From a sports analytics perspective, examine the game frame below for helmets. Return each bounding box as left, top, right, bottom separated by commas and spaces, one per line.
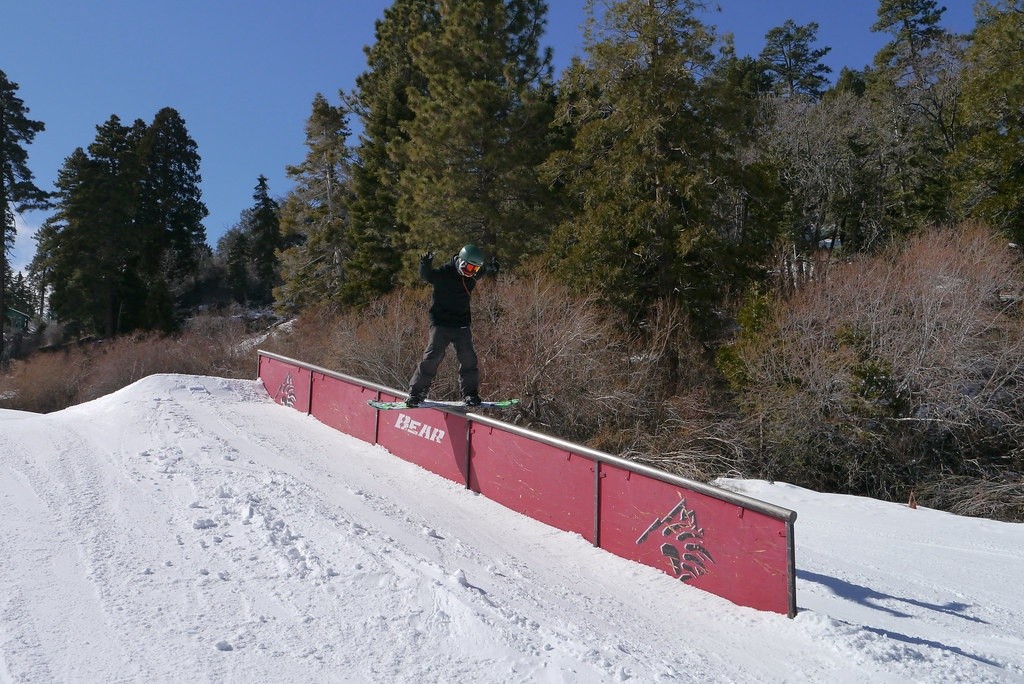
459, 244, 484, 267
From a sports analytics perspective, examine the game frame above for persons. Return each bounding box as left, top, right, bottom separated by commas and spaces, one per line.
405, 244, 500, 407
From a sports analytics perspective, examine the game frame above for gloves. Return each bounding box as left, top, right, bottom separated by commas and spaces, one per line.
420, 252, 437, 264
486, 257, 498, 277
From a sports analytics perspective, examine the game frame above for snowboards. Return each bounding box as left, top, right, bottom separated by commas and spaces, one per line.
367, 399, 520, 412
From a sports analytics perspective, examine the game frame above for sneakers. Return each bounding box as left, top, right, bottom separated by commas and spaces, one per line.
463, 391, 480, 407
407, 395, 423, 407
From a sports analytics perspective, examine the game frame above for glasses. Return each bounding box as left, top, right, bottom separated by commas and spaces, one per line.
465, 263, 480, 273
